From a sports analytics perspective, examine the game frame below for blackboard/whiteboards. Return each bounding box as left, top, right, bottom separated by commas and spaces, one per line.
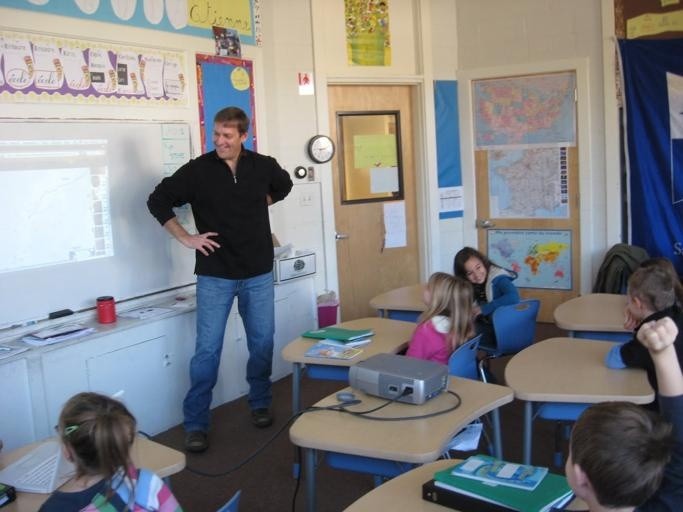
2, 118, 203, 333
268, 184, 328, 299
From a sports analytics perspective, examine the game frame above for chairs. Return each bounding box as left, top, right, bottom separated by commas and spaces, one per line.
216, 489, 246, 512
471, 299, 541, 383
448, 332, 485, 381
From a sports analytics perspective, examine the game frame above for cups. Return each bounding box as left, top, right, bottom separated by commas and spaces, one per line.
97, 296, 115, 323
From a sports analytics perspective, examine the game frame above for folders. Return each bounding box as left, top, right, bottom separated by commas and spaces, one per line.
422, 478, 577, 512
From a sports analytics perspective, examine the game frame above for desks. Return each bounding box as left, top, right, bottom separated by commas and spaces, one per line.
555, 293, 643, 342
280, 317, 421, 483
341, 460, 593, 511
2, 434, 187, 511
506, 336, 654, 468
290, 374, 516, 512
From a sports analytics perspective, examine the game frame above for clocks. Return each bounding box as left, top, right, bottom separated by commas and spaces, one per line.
308, 137, 337, 165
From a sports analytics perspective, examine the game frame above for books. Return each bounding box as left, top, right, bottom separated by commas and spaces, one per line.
297, 322, 379, 360
0, 482, 15, 509
420, 450, 576, 511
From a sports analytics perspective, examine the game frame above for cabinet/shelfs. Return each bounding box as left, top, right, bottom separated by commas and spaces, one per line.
0, 279, 321, 459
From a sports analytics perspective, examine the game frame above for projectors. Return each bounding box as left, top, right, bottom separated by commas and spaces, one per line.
348, 352, 449, 405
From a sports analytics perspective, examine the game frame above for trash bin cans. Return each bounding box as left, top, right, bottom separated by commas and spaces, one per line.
315, 289, 339, 328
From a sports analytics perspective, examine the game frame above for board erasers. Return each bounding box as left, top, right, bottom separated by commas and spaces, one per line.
49, 310, 75, 320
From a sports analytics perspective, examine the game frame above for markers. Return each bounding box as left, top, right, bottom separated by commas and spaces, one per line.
1, 348, 14, 352
12, 320, 41, 329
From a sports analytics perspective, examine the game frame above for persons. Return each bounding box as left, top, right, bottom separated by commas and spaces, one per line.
603, 258, 680, 389
547, 314, 682, 512
36, 389, 183, 512
146, 104, 294, 451
453, 244, 522, 320
406, 271, 476, 366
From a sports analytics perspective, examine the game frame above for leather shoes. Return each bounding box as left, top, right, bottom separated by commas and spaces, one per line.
252, 408, 272, 427
185, 429, 208, 451
